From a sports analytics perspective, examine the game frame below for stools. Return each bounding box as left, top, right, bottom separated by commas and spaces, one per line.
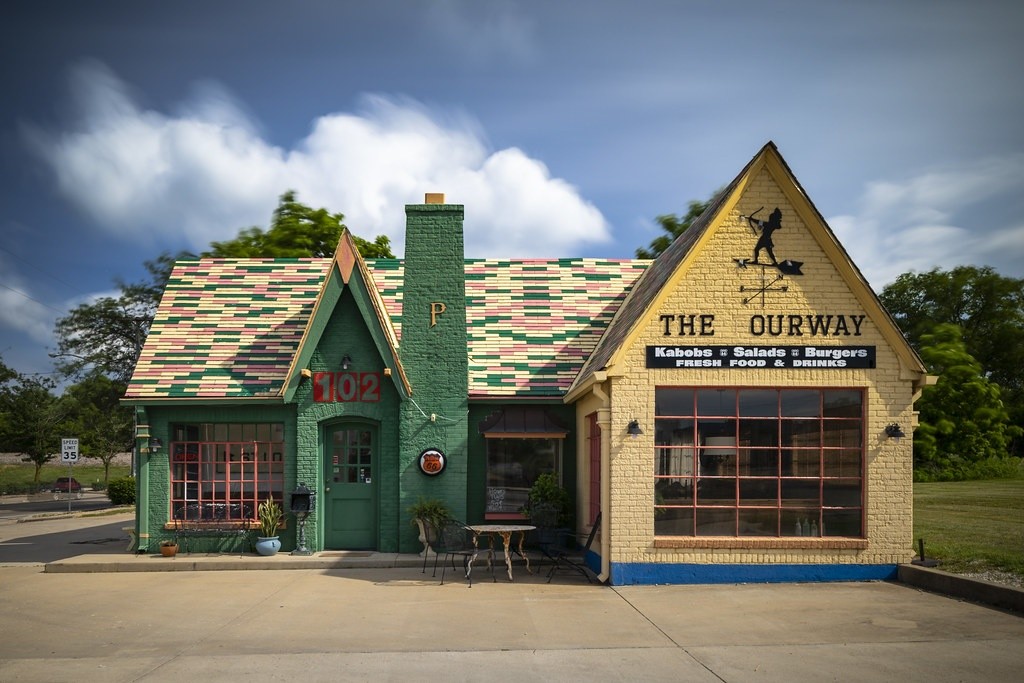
122, 526, 136, 552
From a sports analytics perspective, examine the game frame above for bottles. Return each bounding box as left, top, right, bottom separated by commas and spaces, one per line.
811, 520, 818, 536
795, 517, 801, 536
802, 517, 810, 535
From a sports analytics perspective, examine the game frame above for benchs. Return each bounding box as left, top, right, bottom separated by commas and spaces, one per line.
173, 504, 254, 559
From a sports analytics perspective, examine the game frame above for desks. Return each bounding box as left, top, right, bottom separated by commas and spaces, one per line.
461, 525, 536, 581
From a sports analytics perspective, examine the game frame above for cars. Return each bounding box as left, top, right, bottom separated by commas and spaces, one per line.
55, 478, 81, 492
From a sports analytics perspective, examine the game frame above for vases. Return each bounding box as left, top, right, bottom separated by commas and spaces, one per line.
159, 540, 177, 556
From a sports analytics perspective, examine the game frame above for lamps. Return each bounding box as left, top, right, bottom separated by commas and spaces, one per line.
626, 420, 644, 438
149, 437, 162, 452
340, 354, 353, 369
886, 423, 905, 443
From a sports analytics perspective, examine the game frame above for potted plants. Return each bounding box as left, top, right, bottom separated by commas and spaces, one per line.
255, 496, 290, 556
525, 469, 572, 554
404, 492, 455, 557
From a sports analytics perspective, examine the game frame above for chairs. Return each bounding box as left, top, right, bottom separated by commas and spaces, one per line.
417, 511, 602, 588
